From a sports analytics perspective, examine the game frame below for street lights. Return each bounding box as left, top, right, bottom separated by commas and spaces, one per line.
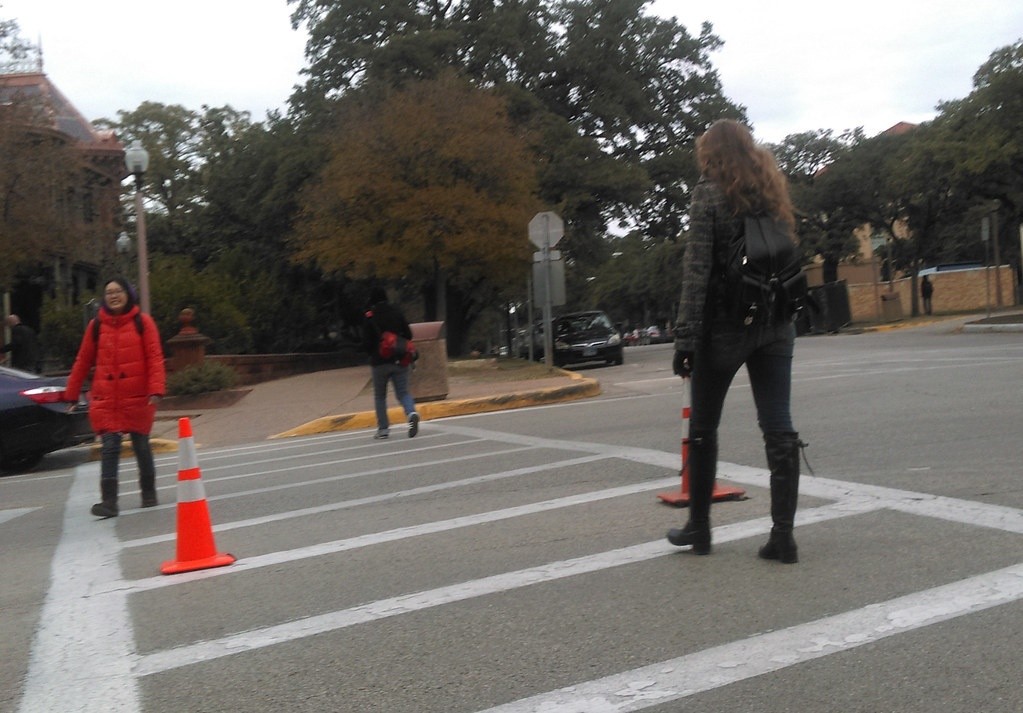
124, 147, 150, 315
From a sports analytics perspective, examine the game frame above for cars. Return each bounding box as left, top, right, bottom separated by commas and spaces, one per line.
514, 310, 674, 368
0, 365, 95, 476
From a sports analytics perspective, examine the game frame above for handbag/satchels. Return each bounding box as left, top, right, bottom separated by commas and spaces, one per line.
379, 332, 419, 368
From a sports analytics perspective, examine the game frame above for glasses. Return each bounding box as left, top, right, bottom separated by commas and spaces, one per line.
106, 289, 125, 296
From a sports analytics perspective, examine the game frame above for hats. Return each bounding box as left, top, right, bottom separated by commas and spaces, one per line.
369, 288, 387, 304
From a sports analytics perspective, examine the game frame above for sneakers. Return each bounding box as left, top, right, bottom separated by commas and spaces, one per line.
408, 412, 419, 439
374, 428, 390, 439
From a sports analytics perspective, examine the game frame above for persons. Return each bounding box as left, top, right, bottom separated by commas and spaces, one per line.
921, 275, 933, 314
359, 287, 419, 439
663, 118, 818, 563
0, 314, 45, 374
62, 278, 171, 514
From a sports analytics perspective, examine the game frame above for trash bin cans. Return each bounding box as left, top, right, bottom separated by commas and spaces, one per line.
881, 291, 903, 323
406, 321, 449, 402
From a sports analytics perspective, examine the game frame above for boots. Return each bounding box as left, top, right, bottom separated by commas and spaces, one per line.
667, 432, 717, 554
758, 432, 814, 564
91, 478, 119, 516
140, 480, 158, 508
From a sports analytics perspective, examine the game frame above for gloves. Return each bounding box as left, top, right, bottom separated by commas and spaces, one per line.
672, 349, 694, 380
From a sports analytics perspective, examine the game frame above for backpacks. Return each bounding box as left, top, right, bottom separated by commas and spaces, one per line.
724, 210, 810, 331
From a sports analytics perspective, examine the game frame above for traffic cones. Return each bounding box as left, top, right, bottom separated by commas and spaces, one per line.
160, 417, 236, 575
657, 376, 746, 506
